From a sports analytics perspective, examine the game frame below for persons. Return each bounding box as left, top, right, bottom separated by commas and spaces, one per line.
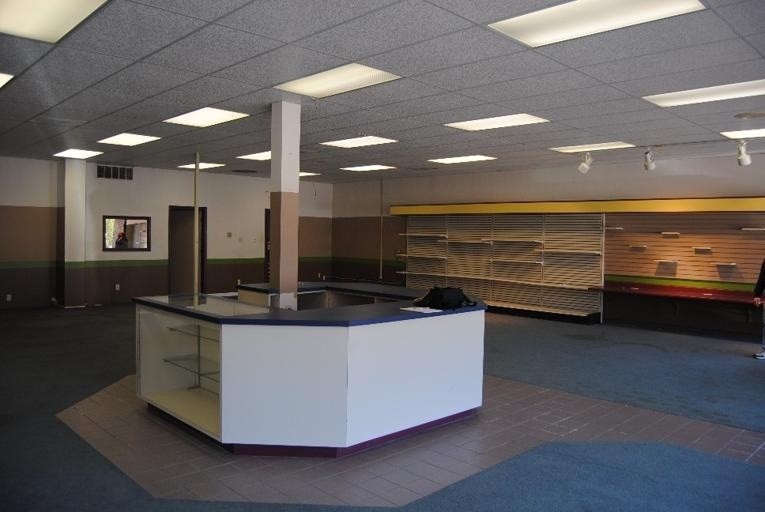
114, 232, 128, 248
749, 257, 765, 360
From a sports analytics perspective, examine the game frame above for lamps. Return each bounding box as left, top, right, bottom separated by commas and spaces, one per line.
577, 139, 764, 175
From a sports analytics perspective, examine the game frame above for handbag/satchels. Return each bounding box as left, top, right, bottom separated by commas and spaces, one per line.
413, 288, 464, 310
